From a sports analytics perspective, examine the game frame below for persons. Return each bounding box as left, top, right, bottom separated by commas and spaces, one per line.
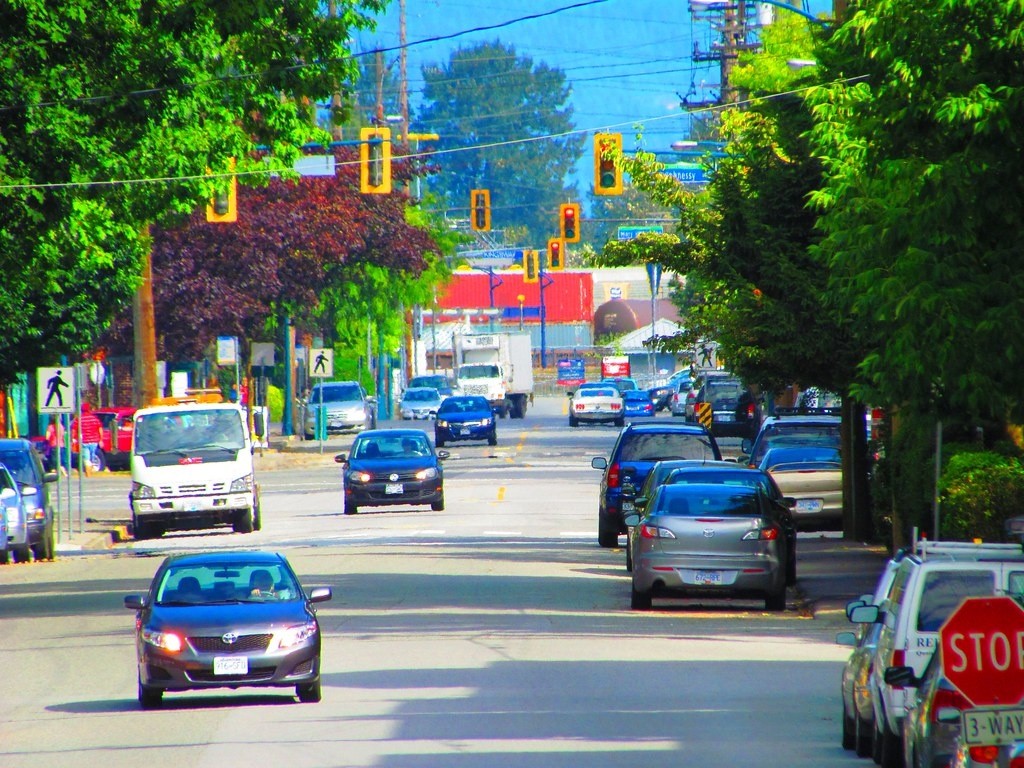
227, 376, 249, 410
396, 439, 423, 457
46, 413, 69, 475
71, 402, 104, 478
250, 572, 280, 601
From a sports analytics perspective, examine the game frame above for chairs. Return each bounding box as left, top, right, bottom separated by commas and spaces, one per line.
250, 570, 276, 594
4, 455, 27, 483
668, 496, 689, 516
178, 576, 204, 599
366, 444, 382, 458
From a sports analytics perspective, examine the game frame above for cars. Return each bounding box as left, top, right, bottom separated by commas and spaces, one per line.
432, 398, 498, 447
399, 373, 456, 420
621, 457, 798, 611
1, 440, 60, 564
649, 368, 847, 530
885, 643, 1024, 768
567, 378, 673, 427
303, 381, 378, 439
125, 555, 330, 706
335, 427, 450, 514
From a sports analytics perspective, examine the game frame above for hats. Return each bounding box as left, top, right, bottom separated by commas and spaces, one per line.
80, 403, 91, 411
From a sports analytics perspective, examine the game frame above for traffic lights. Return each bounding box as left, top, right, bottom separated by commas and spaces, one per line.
557, 204, 579, 240
594, 132, 623, 195
548, 238, 565, 271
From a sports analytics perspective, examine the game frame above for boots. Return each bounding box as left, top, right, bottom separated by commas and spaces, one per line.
85, 466, 93, 478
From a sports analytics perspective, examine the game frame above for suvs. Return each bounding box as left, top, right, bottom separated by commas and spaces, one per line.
837, 539, 1024, 765
593, 417, 739, 547
29, 408, 181, 474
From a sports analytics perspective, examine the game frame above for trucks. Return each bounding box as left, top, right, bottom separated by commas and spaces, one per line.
129, 408, 264, 532
451, 333, 535, 418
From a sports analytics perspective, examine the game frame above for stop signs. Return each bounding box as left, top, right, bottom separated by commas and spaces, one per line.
941, 596, 1024, 708
